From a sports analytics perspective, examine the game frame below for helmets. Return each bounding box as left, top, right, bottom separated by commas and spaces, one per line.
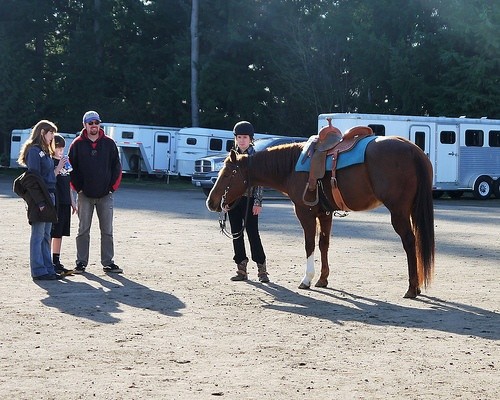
55, 135, 65, 148
233, 121, 254, 140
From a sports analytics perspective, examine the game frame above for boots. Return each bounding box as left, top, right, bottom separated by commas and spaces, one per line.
257, 261, 269, 282
230, 258, 248, 280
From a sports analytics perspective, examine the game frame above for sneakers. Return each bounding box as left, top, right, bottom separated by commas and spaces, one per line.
73, 267, 85, 273
103, 263, 122, 272
53, 261, 73, 277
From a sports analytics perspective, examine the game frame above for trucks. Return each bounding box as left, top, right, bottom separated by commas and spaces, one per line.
99, 123, 181, 182
318, 113, 500, 199
176, 127, 288, 181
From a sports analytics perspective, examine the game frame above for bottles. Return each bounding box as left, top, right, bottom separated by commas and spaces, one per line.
62, 156, 74, 172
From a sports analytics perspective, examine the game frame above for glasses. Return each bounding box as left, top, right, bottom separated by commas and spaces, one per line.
88, 122, 99, 125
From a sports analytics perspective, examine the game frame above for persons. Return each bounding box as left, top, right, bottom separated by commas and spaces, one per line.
13, 119, 64, 282
227, 120, 272, 282
43, 134, 75, 278
127, 148, 143, 180
67, 111, 124, 274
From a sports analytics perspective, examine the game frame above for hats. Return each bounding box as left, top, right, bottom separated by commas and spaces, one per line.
83, 111, 101, 123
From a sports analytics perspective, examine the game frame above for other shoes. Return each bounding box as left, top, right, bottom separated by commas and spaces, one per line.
32, 274, 63, 280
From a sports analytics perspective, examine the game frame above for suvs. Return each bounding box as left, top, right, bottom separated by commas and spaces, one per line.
191, 137, 308, 199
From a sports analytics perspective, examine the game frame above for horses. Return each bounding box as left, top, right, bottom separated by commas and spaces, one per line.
205, 136, 435, 300
